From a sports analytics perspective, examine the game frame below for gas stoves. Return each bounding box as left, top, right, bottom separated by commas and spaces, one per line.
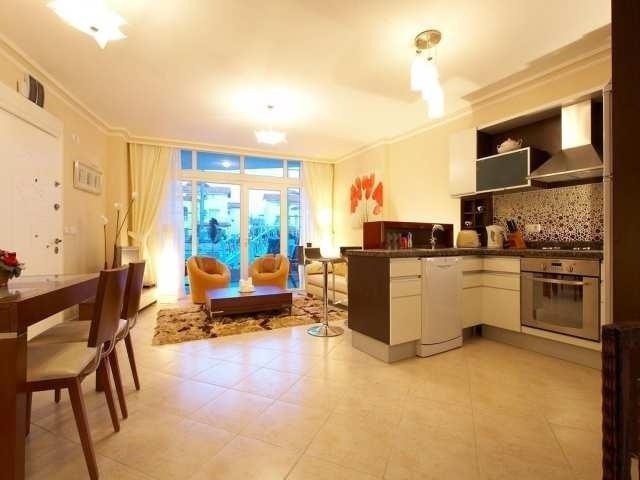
540, 246, 593, 250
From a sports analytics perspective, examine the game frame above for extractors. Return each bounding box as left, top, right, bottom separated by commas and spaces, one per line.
526, 98, 602, 185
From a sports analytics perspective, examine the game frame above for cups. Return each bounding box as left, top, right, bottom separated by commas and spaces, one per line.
239, 276, 253, 292
477, 206, 486, 211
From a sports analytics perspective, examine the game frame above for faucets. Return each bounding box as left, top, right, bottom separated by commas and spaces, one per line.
429, 224, 445, 249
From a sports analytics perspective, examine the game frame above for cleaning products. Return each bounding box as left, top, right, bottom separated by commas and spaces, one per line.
398, 231, 414, 249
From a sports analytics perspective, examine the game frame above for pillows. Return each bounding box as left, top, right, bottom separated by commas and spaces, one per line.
196, 255, 220, 274
258, 254, 280, 274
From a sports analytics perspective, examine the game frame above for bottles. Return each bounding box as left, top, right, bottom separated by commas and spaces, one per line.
385, 232, 412, 249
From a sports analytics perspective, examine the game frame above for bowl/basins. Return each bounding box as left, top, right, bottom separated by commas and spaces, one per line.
465, 222, 472, 225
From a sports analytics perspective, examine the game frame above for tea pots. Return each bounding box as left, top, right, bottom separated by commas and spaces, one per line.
496, 139, 525, 153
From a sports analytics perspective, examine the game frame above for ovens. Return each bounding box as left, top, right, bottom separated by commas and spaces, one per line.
520, 257, 600, 344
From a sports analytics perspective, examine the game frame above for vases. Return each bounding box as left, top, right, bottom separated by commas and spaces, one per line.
0, 268, 15, 286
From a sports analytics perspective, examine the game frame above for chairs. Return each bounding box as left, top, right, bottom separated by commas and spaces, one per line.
25, 255, 147, 424
247, 249, 291, 290
186, 254, 232, 305
18, 260, 131, 480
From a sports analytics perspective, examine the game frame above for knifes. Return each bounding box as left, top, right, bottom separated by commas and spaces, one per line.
506, 219, 517, 233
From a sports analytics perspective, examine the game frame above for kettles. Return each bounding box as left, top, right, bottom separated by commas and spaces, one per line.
485, 226, 504, 249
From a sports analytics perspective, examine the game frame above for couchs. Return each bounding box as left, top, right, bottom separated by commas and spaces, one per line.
303, 256, 349, 310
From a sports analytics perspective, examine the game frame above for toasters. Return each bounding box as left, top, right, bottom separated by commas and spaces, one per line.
455, 230, 482, 247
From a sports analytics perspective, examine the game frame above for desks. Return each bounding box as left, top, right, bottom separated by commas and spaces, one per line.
201, 284, 296, 325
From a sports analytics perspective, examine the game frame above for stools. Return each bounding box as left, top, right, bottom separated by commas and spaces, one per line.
304, 245, 348, 338
338, 245, 363, 262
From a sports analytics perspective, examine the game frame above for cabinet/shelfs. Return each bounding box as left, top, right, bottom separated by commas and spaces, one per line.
481, 249, 522, 350
473, 145, 553, 195
594, 249, 609, 372
343, 251, 423, 364
446, 125, 493, 201
458, 245, 484, 343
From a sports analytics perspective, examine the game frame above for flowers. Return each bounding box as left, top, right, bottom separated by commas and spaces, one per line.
99, 215, 109, 269
1, 248, 26, 279
112, 190, 140, 268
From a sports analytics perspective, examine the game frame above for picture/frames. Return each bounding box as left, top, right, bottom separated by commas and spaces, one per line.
71, 160, 105, 197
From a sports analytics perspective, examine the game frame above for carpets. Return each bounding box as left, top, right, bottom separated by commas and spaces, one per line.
151, 291, 350, 346
